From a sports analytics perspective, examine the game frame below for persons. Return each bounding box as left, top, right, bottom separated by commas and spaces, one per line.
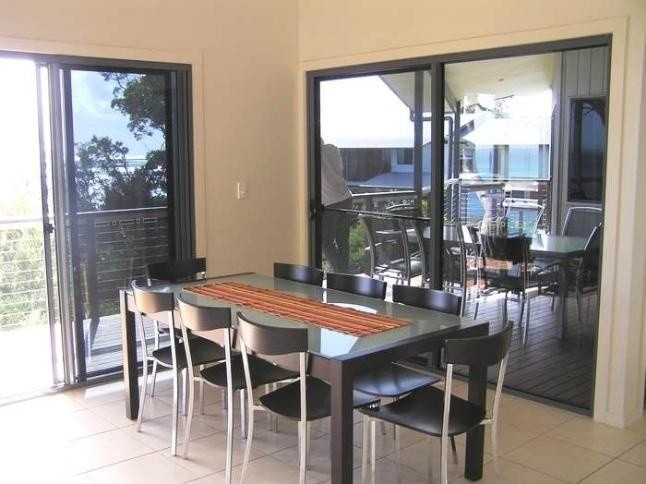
319, 136, 355, 276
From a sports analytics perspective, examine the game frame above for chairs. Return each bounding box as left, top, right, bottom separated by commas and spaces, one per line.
176, 295, 305, 461
357, 319, 516, 484
144, 257, 227, 412
132, 277, 248, 457
337, 282, 465, 470
234, 308, 382, 482
327, 272, 387, 300
355, 196, 608, 349
273, 264, 325, 287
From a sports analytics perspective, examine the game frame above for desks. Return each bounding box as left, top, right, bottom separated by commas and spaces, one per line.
117, 270, 489, 483
